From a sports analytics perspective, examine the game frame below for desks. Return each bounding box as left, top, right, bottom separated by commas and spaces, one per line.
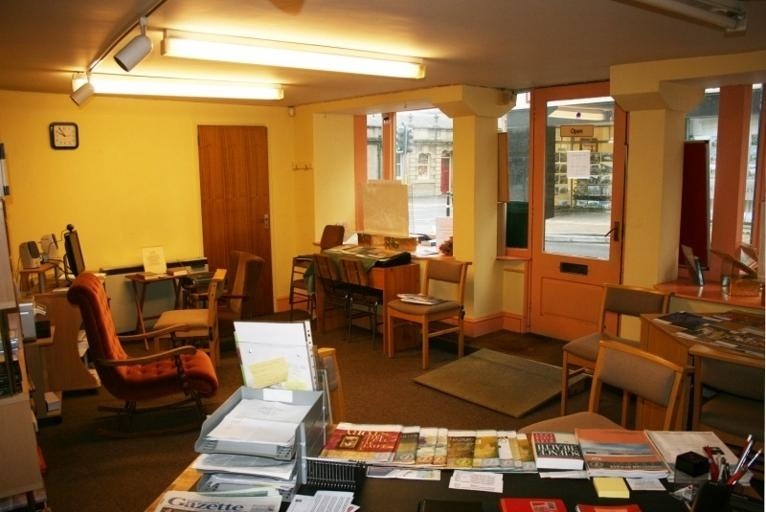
17, 260, 107, 390
1, 329, 45, 498
125, 267, 193, 352
311, 247, 423, 359
636, 308, 766, 454
141, 415, 764, 512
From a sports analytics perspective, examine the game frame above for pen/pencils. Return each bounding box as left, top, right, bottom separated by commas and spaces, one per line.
702, 434, 763, 489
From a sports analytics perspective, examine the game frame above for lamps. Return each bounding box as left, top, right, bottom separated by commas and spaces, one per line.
68, 77, 286, 102
611, 2, 752, 35
157, 24, 427, 83
66, 15, 153, 108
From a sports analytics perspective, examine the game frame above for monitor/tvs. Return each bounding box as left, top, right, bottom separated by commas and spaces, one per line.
63, 230, 86, 283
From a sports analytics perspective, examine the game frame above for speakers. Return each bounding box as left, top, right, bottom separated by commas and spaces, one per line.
40, 233, 59, 260
20, 241, 41, 269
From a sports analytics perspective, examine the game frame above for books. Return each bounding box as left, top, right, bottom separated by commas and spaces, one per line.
166, 267, 189, 278
137, 271, 160, 281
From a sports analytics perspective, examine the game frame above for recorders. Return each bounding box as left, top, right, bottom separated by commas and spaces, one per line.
181, 272, 230, 294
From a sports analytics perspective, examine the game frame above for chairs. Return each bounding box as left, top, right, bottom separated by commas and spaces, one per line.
518, 337, 689, 437
187, 249, 252, 333
67, 270, 219, 434
316, 253, 369, 339
339, 256, 383, 351
385, 258, 474, 370
288, 222, 347, 324
557, 281, 675, 420
151, 269, 227, 372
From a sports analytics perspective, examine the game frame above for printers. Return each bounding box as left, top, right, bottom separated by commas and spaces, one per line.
19, 295, 52, 341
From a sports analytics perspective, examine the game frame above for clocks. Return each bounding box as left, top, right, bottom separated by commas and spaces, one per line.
47, 120, 80, 150
